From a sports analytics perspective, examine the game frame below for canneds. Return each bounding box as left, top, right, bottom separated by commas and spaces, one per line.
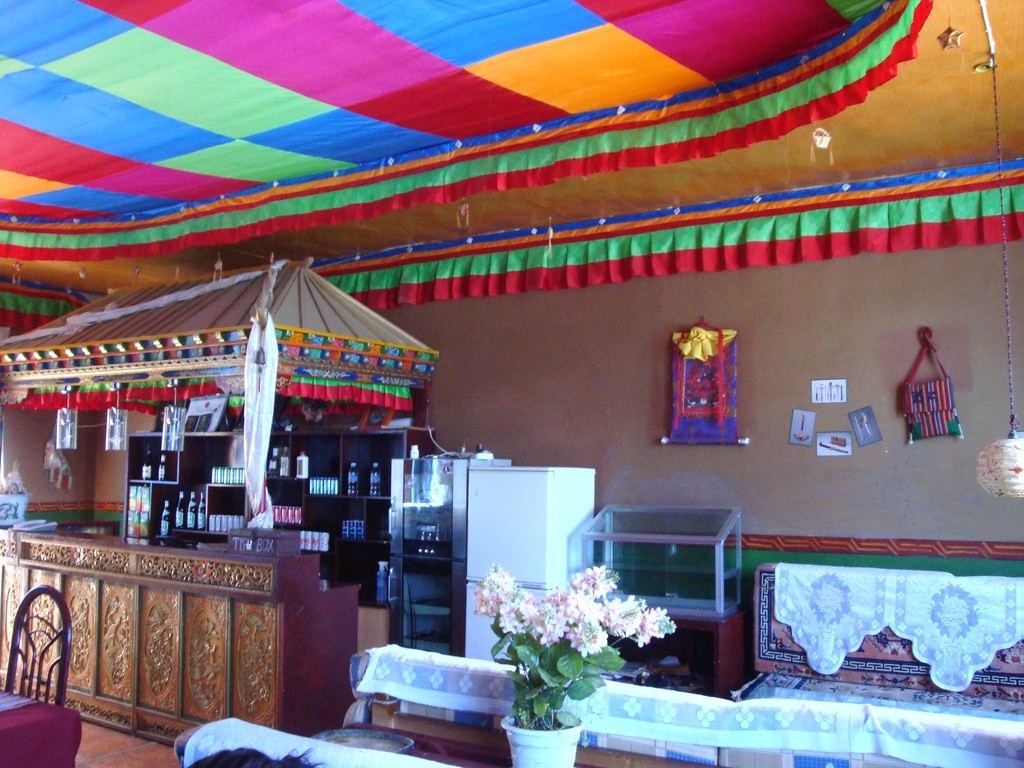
341, 520, 364, 539
299, 531, 330, 552
271, 505, 302, 525
211, 467, 246, 484
309, 476, 339, 495
208, 514, 244, 533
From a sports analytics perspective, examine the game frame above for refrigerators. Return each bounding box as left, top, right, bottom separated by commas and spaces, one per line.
466, 466, 595, 661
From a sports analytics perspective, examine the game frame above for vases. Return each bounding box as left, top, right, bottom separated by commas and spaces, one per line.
498, 711, 584, 768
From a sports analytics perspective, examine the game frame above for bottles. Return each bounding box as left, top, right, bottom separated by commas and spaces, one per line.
347, 462, 359, 497
376, 561, 389, 603
296, 450, 309, 479
280, 446, 289, 477
141, 442, 152, 481
369, 461, 381, 497
266, 447, 278, 477
175, 491, 186, 529
187, 491, 197, 529
197, 492, 205, 531
159, 500, 170, 536
157, 450, 167, 481
409, 444, 420, 458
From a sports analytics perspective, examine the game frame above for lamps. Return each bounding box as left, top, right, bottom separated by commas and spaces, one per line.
55, 378, 188, 453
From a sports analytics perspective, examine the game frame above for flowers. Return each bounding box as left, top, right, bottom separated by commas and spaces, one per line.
472, 559, 678, 732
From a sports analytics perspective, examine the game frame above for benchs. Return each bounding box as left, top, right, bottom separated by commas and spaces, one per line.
348, 646, 1021, 768
734, 560, 1024, 723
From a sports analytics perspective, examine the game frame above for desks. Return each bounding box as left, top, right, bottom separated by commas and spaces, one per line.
0, 689, 83, 768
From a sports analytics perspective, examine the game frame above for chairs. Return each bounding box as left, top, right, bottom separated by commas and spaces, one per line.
4, 583, 73, 708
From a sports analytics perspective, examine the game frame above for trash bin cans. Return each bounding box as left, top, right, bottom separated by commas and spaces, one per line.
308, 728, 415, 756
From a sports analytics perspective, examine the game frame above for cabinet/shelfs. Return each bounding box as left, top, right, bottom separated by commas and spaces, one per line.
120, 428, 435, 610
605, 611, 747, 700
